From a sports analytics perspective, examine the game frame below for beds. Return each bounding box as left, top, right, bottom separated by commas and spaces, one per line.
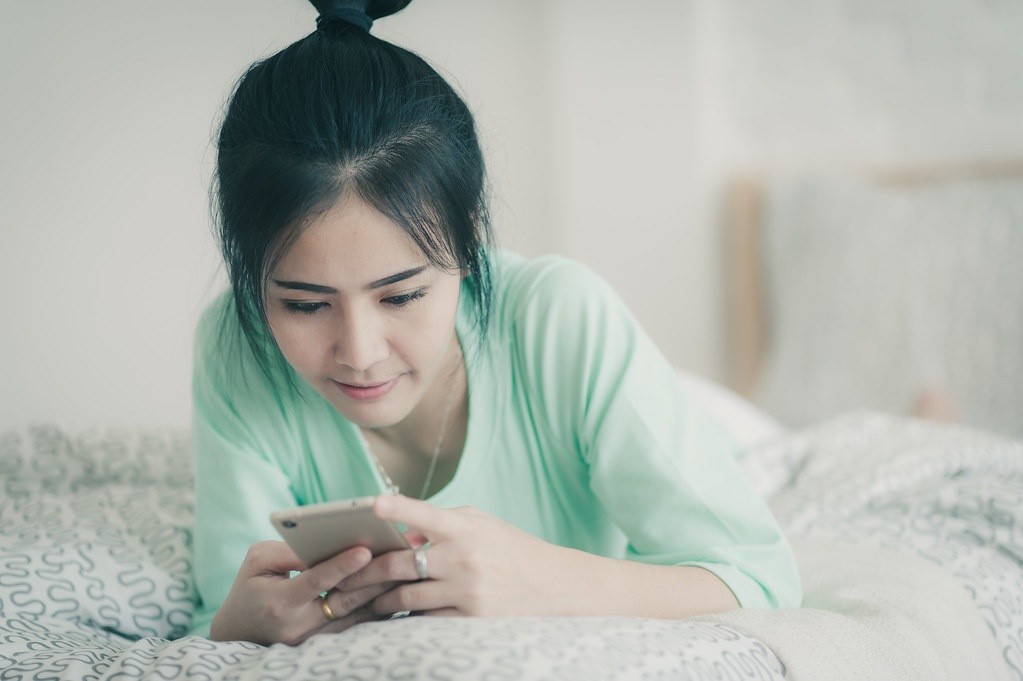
0, 407, 1023, 681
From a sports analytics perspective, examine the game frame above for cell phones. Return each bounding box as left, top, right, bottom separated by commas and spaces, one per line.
271, 495, 414, 596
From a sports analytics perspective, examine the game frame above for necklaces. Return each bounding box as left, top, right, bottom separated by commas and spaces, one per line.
365, 397, 456, 499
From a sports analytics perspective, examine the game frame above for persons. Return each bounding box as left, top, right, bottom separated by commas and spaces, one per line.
189, 0, 804, 647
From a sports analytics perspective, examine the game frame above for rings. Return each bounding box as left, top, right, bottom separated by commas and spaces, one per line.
415, 548, 428, 578
321, 598, 338, 621
